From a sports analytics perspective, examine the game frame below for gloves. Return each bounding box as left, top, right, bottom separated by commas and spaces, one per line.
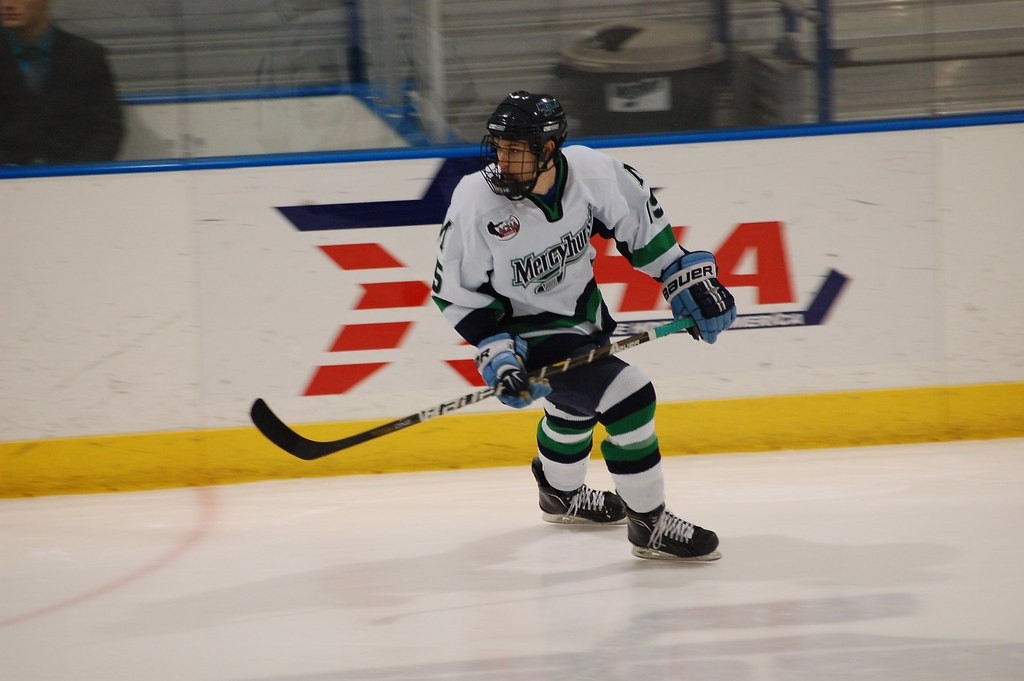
660, 250, 738, 345
475, 333, 553, 409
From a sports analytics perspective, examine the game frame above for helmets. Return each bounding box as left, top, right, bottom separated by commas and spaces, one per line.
479, 91, 569, 196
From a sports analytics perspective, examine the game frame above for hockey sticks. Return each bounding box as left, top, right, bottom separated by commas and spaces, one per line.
250, 315, 696, 461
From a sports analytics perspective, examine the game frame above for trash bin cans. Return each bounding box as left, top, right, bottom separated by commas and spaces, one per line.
556, 15, 732, 135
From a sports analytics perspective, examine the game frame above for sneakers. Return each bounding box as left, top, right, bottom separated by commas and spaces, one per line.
532, 457, 629, 526
615, 490, 721, 561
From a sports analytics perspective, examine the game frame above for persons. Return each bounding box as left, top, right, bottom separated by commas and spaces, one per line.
0, 0, 127, 166
430, 90, 737, 563
